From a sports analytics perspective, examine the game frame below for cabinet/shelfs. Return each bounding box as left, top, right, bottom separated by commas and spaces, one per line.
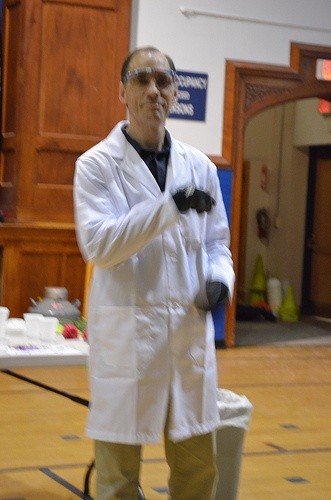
0, 0, 130, 320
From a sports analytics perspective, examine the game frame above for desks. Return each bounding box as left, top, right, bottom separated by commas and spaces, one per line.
0, 332, 145, 500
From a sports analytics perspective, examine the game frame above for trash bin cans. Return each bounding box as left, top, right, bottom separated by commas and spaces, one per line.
215, 389, 254, 500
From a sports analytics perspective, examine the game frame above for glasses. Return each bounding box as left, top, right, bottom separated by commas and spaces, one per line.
123, 68, 178, 88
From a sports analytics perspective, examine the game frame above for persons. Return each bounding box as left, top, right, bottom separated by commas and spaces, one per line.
72, 47, 233, 500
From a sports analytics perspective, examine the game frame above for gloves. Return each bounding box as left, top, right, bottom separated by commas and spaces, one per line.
174, 186, 216, 213
195, 281, 227, 310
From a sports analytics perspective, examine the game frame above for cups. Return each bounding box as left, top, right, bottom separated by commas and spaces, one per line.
23, 312, 43, 339
40, 317, 59, 342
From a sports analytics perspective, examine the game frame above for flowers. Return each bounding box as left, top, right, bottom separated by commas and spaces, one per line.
62, 323, 87, 341
